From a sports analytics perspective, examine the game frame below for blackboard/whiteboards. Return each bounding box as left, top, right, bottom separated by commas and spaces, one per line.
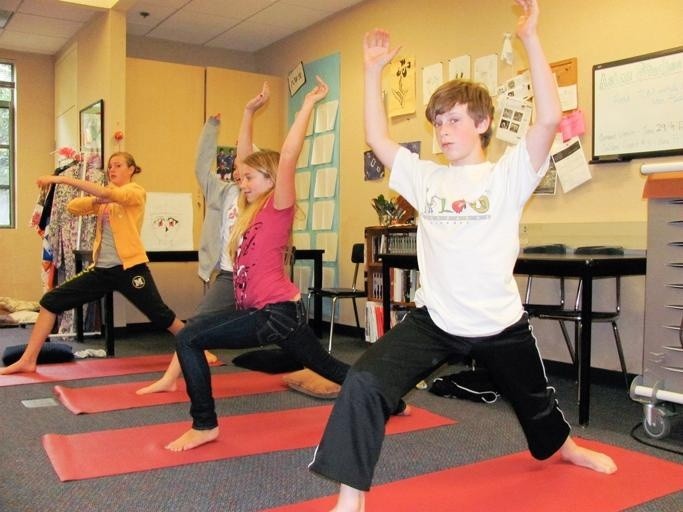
588, 46, 683, 164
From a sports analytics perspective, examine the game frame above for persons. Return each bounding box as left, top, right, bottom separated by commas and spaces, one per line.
164, 80, 412, 454
134, 110, 249, 396
304, 0, 618, 511
0, 152, 219, 376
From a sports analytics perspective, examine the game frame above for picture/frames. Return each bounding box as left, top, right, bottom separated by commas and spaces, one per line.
79, 99, 105, 171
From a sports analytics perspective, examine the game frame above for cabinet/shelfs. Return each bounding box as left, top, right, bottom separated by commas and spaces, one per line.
365, 225, 419, 348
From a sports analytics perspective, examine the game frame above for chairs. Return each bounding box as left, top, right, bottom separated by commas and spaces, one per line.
514, 245, 628, 391
308, 243, 366, 354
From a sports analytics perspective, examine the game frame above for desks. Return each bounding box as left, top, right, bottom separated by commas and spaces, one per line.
376, 252, 647, 429
